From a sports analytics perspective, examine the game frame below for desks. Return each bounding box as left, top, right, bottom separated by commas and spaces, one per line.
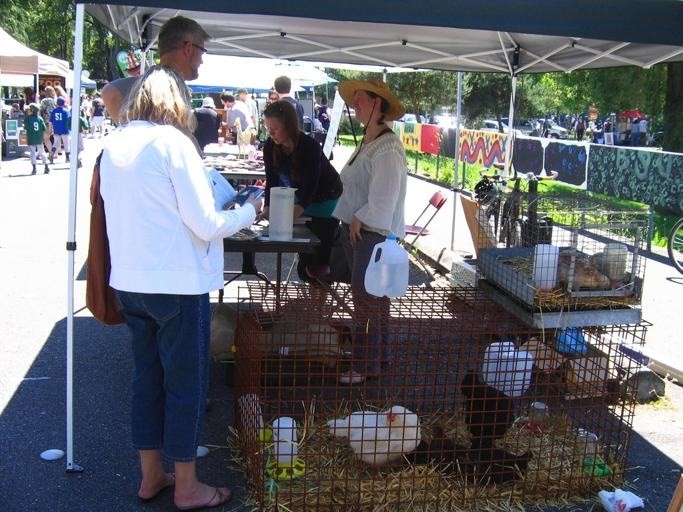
204, 159, 266, 190
204, 142, 255, 158
220, 205, 321, 306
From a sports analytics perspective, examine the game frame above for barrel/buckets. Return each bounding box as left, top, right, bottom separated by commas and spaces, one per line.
362, 233, 410, 299
482, 341, 533, 397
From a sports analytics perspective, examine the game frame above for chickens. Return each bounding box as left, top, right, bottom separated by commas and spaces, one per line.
428, 422, 469, 474
461, 424, 533, 487
459, 373, 515, 438
326, 405, 422, 465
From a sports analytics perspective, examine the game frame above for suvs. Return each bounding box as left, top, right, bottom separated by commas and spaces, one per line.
532, 119, 568, 139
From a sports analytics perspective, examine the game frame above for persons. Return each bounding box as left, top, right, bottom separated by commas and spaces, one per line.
255, 100, 344, 313
315, 103, 332, 160
11, 86, 109, 175
539, 115, 648, 148
192, 96, 220, 150
274, 75, 304, 133
237, 87, 259, 137
100, 65, 263, 512
331, 79, 407, 384
268, 90, 278, 104
220, 91, 256, 146
101, 16, 210, 127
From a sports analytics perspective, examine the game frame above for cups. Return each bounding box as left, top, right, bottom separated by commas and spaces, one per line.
269, 186, 299, 242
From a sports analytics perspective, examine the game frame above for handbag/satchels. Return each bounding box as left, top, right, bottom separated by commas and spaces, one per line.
84, 148, 125, 325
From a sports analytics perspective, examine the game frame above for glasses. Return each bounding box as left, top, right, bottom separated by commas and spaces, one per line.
184, 40, 208, 55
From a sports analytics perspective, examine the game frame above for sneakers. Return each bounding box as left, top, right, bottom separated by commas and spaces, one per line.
339, 368, 368, 384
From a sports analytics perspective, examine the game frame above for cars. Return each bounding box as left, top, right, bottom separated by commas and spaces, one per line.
474, 120, 523, 137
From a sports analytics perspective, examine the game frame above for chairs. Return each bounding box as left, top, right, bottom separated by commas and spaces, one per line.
397, 190, 447, 281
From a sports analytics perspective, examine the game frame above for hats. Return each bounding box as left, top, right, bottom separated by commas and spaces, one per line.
338, 77, 407, 121
57, 97, 65, 106
28, 102, 40, 113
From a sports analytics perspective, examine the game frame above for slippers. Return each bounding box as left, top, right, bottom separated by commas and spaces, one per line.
176, 486, 233, 511
137, 471, 175, 502
